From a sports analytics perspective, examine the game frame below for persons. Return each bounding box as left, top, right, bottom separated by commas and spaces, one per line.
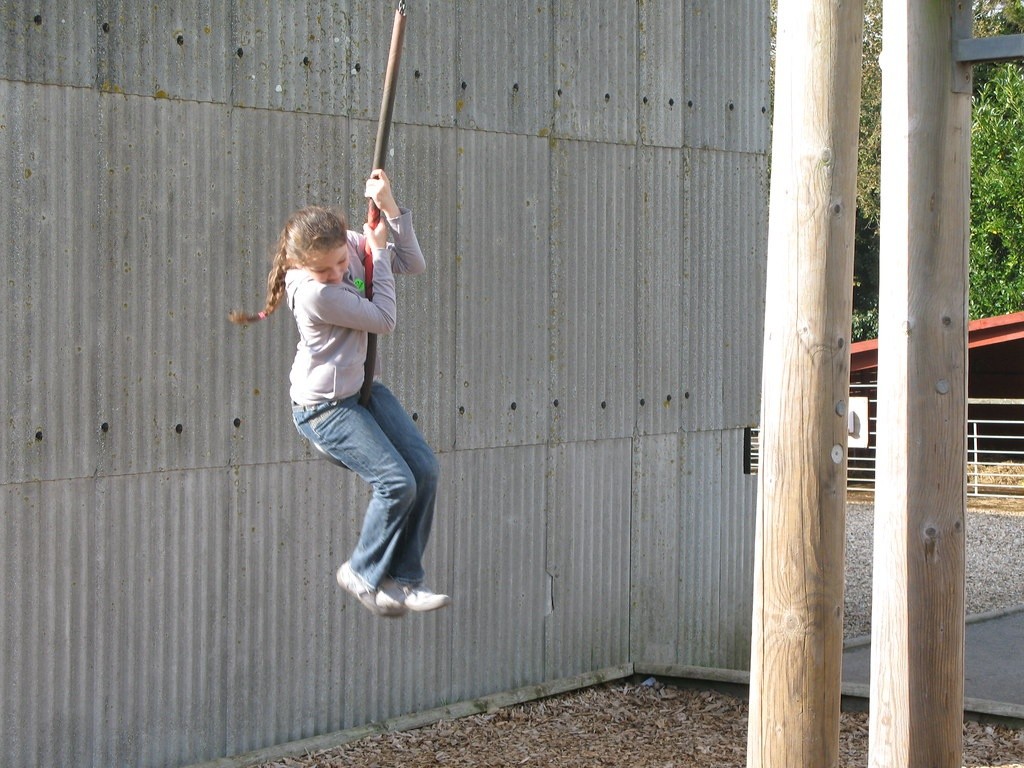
226, 168, 448, 620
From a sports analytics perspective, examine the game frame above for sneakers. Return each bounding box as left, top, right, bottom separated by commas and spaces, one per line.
337, 558, 407, 616
381, 577, 450, 611
